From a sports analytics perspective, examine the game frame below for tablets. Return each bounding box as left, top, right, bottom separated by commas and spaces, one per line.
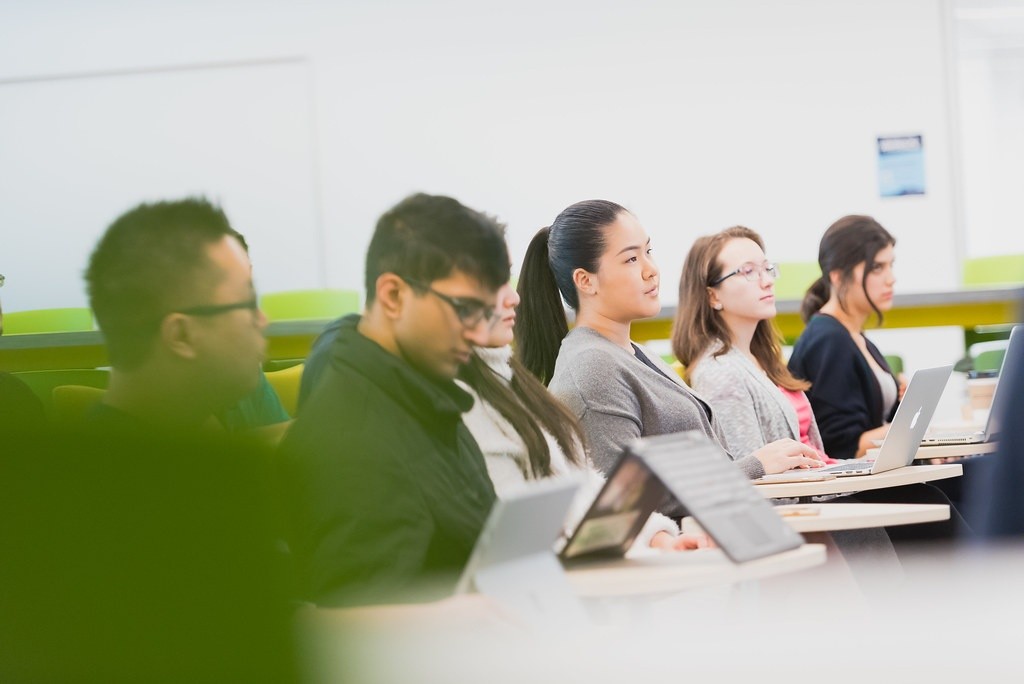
456, 469, 590, 593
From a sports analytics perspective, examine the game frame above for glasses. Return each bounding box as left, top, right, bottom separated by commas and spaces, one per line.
172, 294, 258, 315
710, 261, 777, 286
399, 275, 498, 330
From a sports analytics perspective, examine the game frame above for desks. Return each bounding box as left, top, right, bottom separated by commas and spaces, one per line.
679, 503, 952, 535
757, 463, 967, 502
469, 545, 828, 618
868, 438, 1004, 463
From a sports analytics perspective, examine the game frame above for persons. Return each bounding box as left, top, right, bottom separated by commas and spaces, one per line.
0, 194, 722, 684
513, 200, 978, 592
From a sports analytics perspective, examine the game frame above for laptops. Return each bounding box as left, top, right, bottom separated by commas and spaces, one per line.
918, 325, 1024, 446
780, 366, 952, 478
